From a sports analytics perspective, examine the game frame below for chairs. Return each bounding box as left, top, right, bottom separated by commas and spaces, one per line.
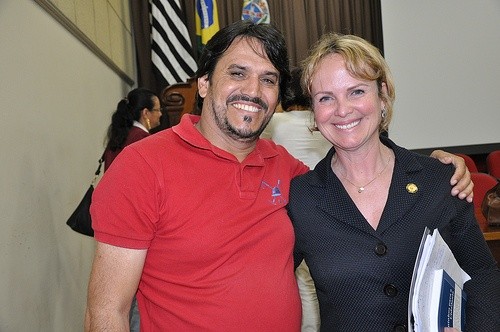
456, 151, 500, 232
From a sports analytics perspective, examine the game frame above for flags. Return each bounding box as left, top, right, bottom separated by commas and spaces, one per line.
195, 0, 220, 63
149, 0, 200, 89
241, 0, 270, 25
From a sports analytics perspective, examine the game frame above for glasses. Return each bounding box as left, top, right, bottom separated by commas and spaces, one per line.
152, 108, 163, 112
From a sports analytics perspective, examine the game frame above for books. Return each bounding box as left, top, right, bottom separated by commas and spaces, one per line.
408, 226, 472, 332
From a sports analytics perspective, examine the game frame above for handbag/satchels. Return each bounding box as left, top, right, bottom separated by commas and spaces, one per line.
66, 184, 94, 237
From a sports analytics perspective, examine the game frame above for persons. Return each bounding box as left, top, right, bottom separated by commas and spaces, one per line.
84, 23, 474, 332
104, 87, 162, 332
285, 34, 500, 332
258, 65, 334, 171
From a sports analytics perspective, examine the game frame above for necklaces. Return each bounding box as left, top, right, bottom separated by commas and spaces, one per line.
334, 148, 390, 193
